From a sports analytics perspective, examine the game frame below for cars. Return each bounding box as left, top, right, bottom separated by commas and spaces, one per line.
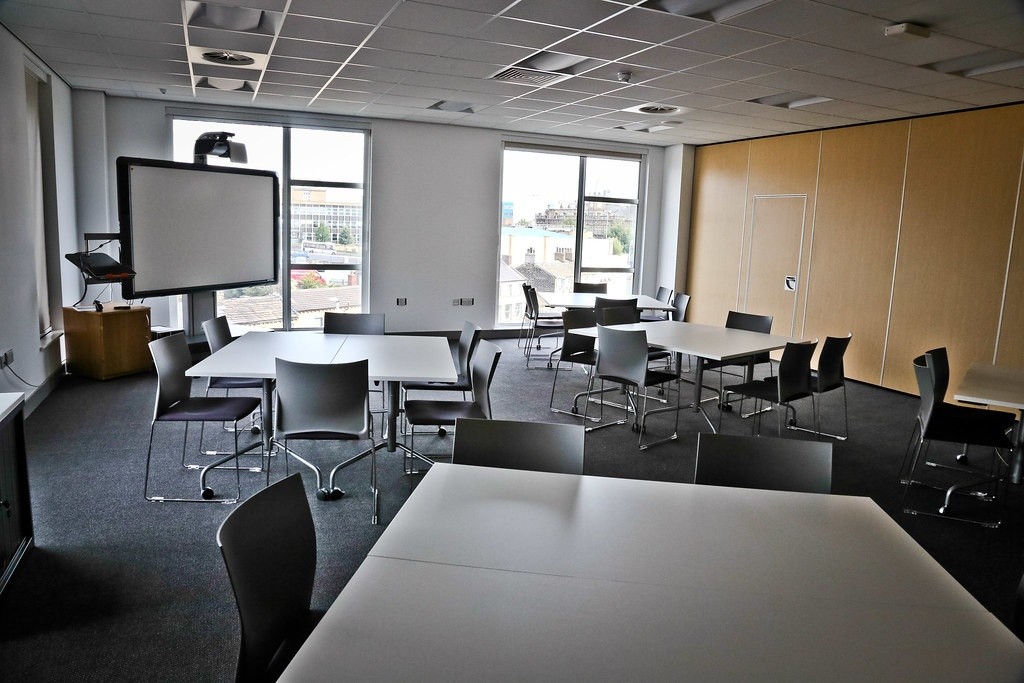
291, 270, 325, 287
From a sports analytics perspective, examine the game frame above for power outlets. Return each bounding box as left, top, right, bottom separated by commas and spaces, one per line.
397, 298, 407, 306
460, 298, 474, 306
452, 299, 459, 306
0, 348, 14, 370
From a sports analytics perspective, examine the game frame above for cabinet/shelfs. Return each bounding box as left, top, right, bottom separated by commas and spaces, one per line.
61, 303, 151, 380
0, 392, 35, 594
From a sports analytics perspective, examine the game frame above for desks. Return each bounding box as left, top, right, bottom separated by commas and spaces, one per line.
185, 332, 348, 502
640, 328, 811, 434
536, 292, 677, 368
273, 555, 1024, 683
330, 334, 458, 501
568, 321, 725, 432
938, 360, 1024, 515
366, 463, 990, 612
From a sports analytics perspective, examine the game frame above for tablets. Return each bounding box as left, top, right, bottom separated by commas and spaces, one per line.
75, 253, 136, 278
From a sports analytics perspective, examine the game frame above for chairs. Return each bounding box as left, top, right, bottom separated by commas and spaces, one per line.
701, 311, 773, 409
897, 355, 1015, 530
906, 348, 1019, 503
526, 288, 573, 372
401, 320, 482, 459
694, 433, 832, 495
265, 357, 379, 525
583, 322, 681, 451
216, 471, 328, 683
323, 313, 386, 439
718, 336, 818, 440
199, 315, 279, 457
594, 296, 639, 327
550, 308, 639, 424
403, 339, 503, 495
144, 332, 263, 505
603, 308, 671, 396
452, 417, 585, 475
640, 287, 673, 322
518, 283, 562, 357
640, 293, 691, 372
758, 331, 853, 443
